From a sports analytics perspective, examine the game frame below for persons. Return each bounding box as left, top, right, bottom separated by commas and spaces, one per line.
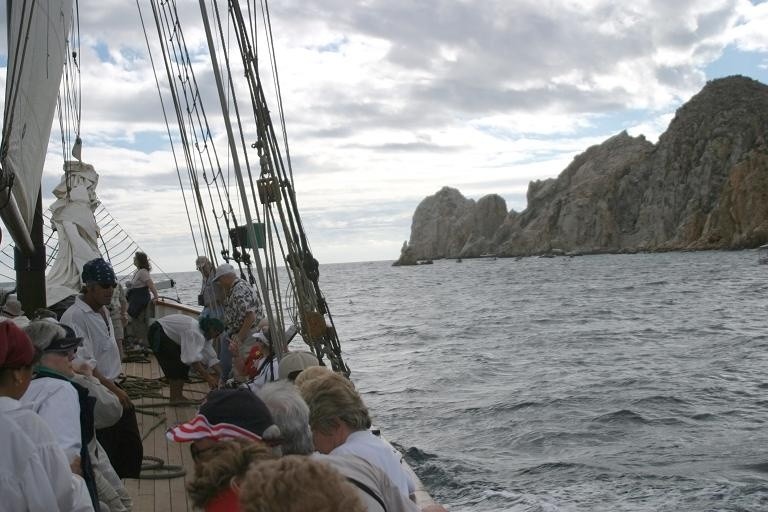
196, 256, 264, 379
125, 253, 159, 347
59, 258, 143, 477
0, 286, 133, 510
147, 314, 224, 406
166, 327, 446, 512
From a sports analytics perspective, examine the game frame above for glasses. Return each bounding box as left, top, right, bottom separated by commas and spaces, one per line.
97, 282, 118, 289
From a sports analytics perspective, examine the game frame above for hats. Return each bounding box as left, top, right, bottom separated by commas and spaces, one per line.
277, 351, 319, 383
195, 256, 236, 282
165, 389, 273, 444
0, 298, 86, 368
82, 257, 117, 282
